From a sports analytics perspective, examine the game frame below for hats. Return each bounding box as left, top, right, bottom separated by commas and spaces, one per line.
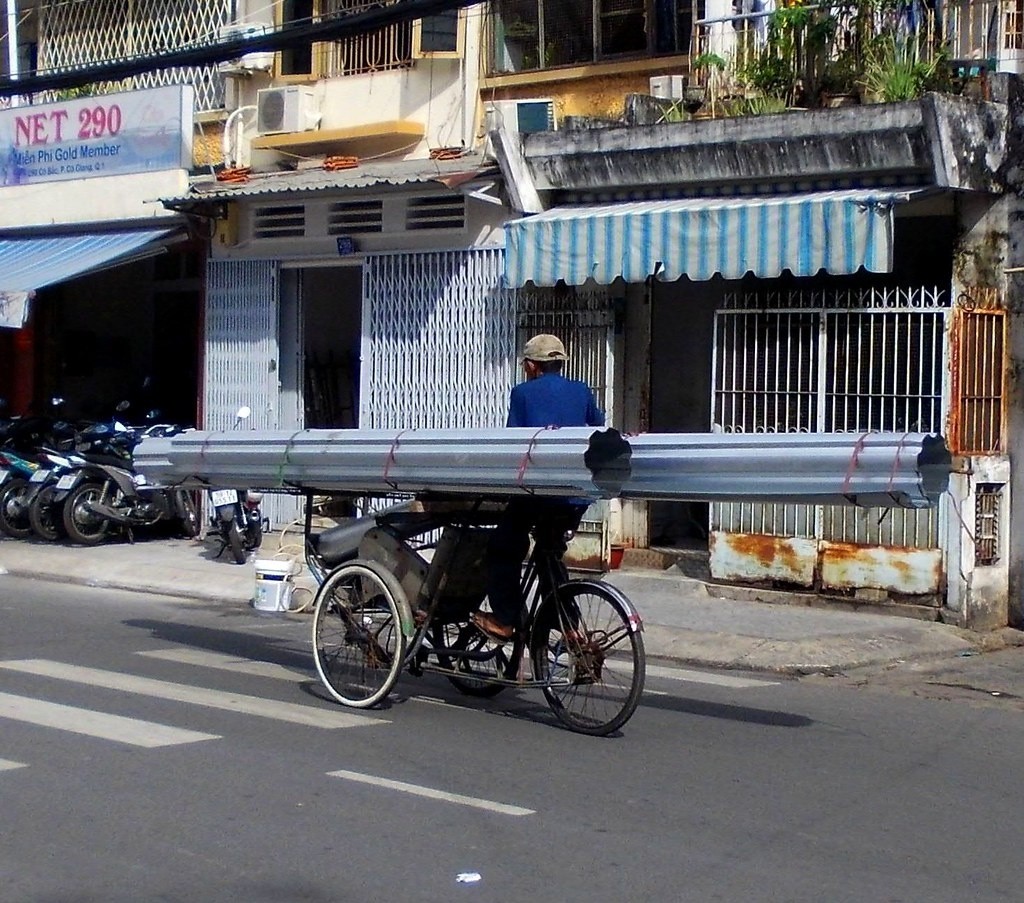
519, 333, 570, 362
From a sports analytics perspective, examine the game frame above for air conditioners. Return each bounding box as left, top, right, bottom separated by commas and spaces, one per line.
650, 74, 689, 99
220, 24, 275, 77
483, 98, 556, 141
254, 86, 321, 138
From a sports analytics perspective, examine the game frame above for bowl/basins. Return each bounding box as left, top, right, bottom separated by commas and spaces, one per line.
611, 544, 624, 569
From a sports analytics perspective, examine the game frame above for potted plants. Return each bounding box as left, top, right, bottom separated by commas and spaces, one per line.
670, 1, 964, 122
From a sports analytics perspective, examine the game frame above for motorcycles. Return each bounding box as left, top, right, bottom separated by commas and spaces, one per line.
181, 406, 264, 565
0, 377, 198, 566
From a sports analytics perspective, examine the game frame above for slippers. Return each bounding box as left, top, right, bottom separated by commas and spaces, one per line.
553, 632, 582, 651
469, 608, 508, 645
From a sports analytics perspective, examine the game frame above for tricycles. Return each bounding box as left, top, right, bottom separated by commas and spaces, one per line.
292, 489, 648, 737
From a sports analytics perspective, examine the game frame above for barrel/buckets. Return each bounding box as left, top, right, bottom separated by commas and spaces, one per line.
253, 559, 294, 613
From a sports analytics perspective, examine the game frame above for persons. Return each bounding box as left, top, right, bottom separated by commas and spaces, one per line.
465, 331, 607, 653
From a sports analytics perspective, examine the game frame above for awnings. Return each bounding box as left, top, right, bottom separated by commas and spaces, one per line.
0, 220, 188, 331
502, 184, 940, 289
